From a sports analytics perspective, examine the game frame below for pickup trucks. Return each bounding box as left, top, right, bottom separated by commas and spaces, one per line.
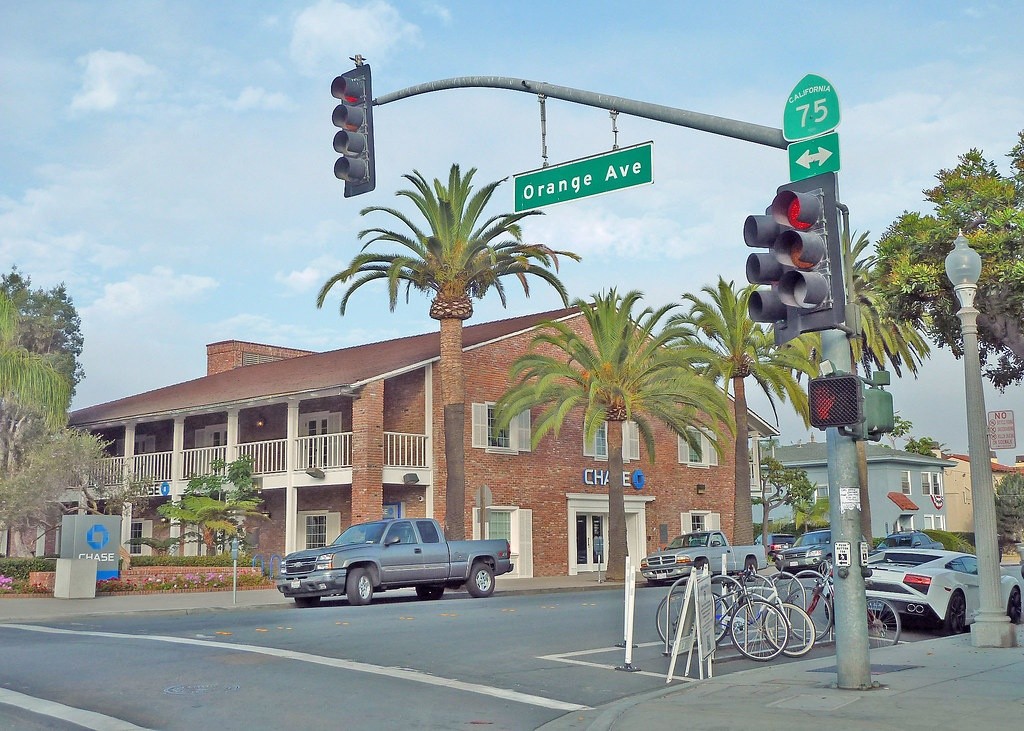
274, 517, 514, 610
640, 530, 768, 587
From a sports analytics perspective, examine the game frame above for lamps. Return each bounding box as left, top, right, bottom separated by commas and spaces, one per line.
256, 413, 265, 432
403, 473, 420, 486
261, 505, 272, 519
253, 477, 263, 489
305, 467, 325, 479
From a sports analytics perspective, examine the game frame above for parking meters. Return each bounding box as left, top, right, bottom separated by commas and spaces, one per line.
231, 538, 239, 604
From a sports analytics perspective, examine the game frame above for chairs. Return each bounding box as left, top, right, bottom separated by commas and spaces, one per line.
691, 540, 701, 546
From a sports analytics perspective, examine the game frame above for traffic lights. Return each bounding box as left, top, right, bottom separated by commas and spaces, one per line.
807, 374, 862, 427
330, 64, 376, 198
771, 171, 845, 333
744, 204, 799, 346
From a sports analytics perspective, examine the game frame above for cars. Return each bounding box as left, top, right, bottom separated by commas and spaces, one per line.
825, 546, 1024, 635
754, 532, 797, 561
868, 529, 945, 556
774, 529, 832, 577
655, 551, 902, 662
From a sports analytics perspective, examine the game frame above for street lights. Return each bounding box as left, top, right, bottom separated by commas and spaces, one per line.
943, 227, 1017, 648
